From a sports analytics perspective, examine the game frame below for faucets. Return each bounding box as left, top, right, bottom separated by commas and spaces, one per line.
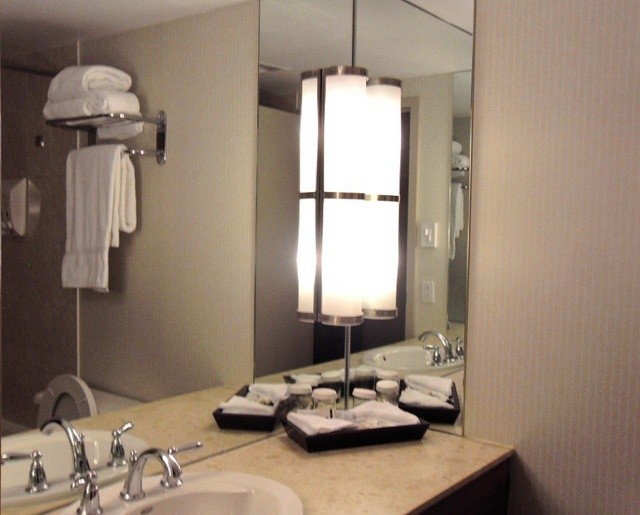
417, 330, 455, 361
119, 448, 181, 499
39, 419, 89, 473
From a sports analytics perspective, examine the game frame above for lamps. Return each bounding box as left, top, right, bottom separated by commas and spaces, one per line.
295, 71, 321, 323
318, 64, 369, 327
367, 76, 403, 321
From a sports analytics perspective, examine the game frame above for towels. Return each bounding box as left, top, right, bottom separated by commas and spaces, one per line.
451, 154, 469, 167
399, 373, 455, 411
287, 399, 421, 439
219, 380, 288, 416
37, 96, 150, 140
451, 141, 462, 155
450, 183, 464, 261
62, 144, 140, 295
45, 63, 141, 96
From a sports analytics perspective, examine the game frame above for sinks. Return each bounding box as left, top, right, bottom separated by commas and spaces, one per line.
67, 471, 304, 515
0, 428, 147, 500
365, 344, 459, 373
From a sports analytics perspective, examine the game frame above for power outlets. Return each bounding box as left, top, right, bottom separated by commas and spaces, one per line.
422, 281, 435, 304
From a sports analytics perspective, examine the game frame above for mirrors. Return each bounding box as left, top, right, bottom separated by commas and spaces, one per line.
346, 0, 476, 437
2, 1, 347, 514
254, 1, 348, 408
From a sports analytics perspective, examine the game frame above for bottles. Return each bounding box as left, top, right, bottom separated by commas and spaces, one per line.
289, 385, 311, 412
376, 379, 398, 406
352, 388, 377, 410
355, 365, 374, 379
376, 370, 398, 378
312, 387, 337, 420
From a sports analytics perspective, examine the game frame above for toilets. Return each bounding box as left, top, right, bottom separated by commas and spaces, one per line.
33, 373, 145, 427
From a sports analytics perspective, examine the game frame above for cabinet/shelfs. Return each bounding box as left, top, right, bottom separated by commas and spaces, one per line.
452, 166, 469, 191
48, 116, 172, 163
